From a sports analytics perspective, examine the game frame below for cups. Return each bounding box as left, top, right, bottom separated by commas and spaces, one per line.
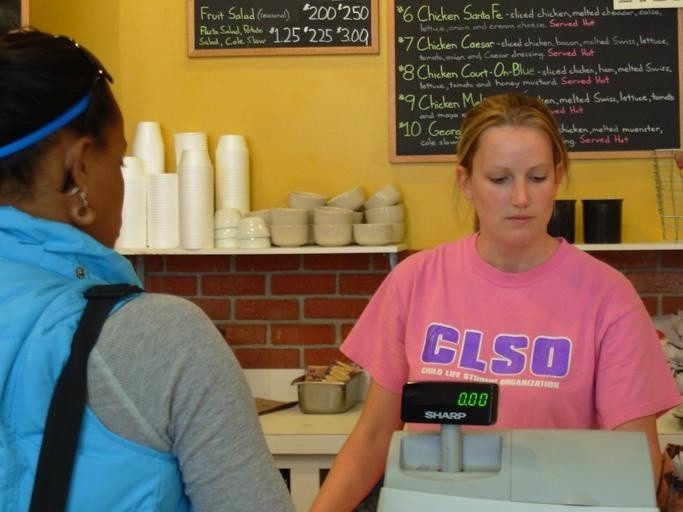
545, 198, 577, 246
581, 198, 623, 244
111, 121, 250, 252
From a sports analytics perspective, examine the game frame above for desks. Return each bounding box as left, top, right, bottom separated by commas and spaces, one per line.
257, 394, 683, 512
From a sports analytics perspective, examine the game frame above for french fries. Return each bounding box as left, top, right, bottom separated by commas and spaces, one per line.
322, 359, 365, 385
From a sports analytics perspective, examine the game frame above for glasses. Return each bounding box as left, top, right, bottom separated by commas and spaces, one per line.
0, 26, 114, 156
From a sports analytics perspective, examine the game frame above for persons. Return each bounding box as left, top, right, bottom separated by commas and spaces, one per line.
1, 25, 295, 510
309, 90, 681, 511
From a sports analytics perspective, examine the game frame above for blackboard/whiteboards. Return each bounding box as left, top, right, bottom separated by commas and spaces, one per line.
386, 0, 683, 164
187, 0, 380, 59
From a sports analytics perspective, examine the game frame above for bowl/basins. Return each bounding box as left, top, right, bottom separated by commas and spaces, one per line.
238, 216, 270, 250
269, 181, 407, 246
